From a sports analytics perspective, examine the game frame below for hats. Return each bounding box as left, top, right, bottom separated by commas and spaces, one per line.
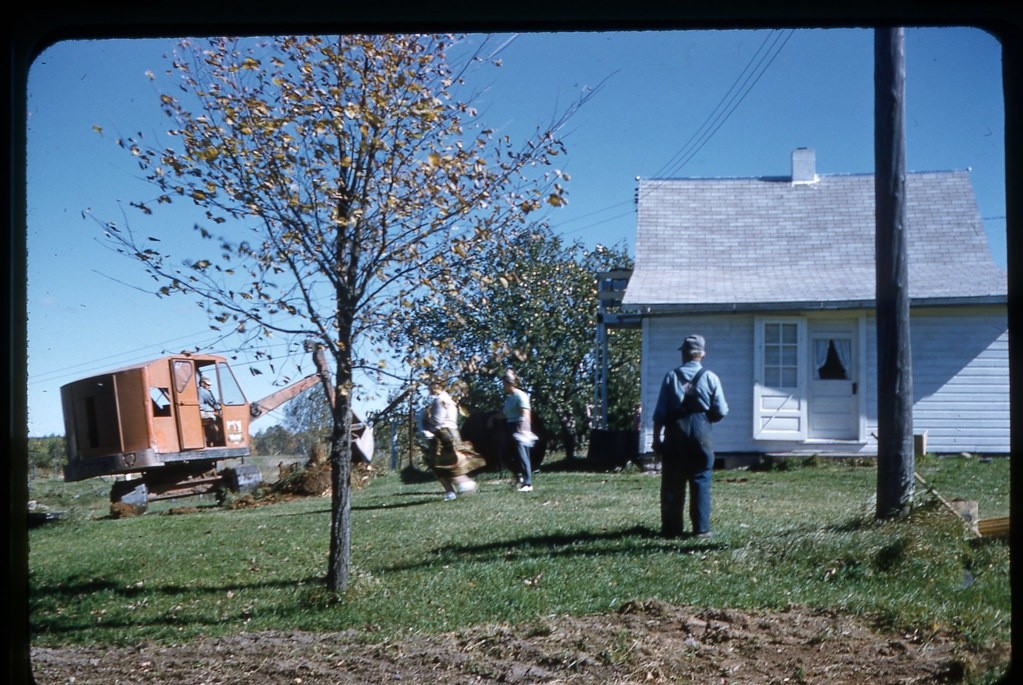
200, 376, 212, 385
505, 371, 515, 384
677, 334, 705, 351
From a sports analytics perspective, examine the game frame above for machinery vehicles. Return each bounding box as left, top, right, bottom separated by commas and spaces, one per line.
58, 342, 376, 519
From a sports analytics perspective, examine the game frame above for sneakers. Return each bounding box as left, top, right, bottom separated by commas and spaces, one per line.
518, 485, 533, 491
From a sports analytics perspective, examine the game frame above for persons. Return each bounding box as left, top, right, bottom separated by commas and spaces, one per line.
502, 372, 539, 492
198, 376, 222, 422
417, 382, 486, 501
650, 335, 728, 538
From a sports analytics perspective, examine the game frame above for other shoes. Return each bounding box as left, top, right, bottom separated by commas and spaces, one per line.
445, 492, 456, 499
695, 531, 711, 538
